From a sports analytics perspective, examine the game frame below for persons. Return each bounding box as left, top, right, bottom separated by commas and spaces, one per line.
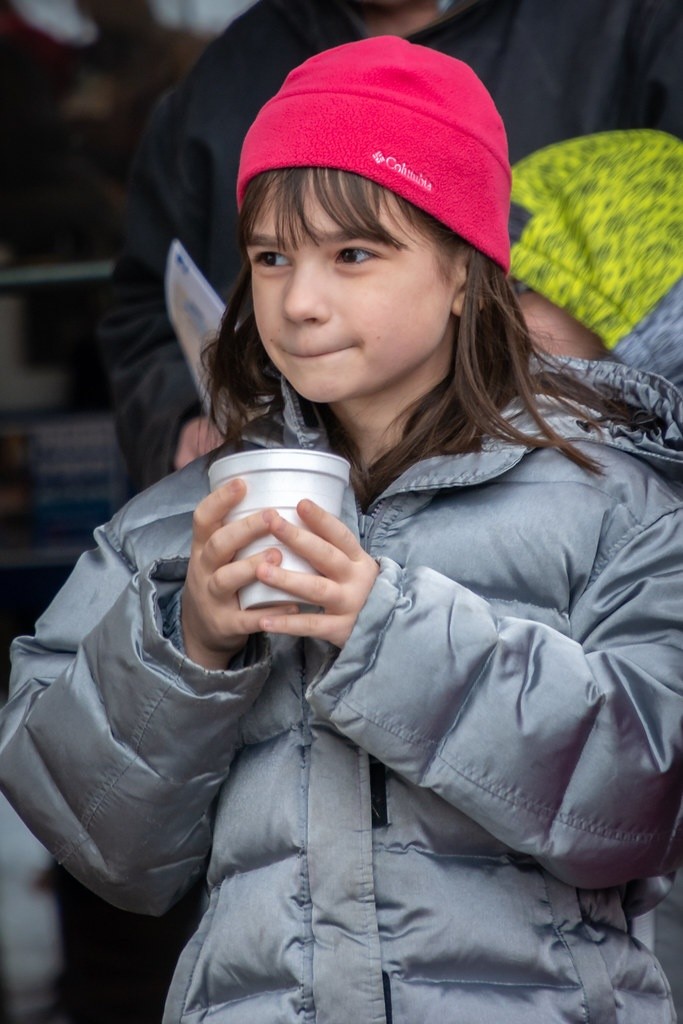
0, 0, 683, 1024
509, 129, 683, 390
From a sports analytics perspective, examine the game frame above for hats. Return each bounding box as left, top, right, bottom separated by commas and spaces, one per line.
236, 34, 512, 271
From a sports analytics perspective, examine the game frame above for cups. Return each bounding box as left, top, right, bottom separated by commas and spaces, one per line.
208, 448, 351, 618
